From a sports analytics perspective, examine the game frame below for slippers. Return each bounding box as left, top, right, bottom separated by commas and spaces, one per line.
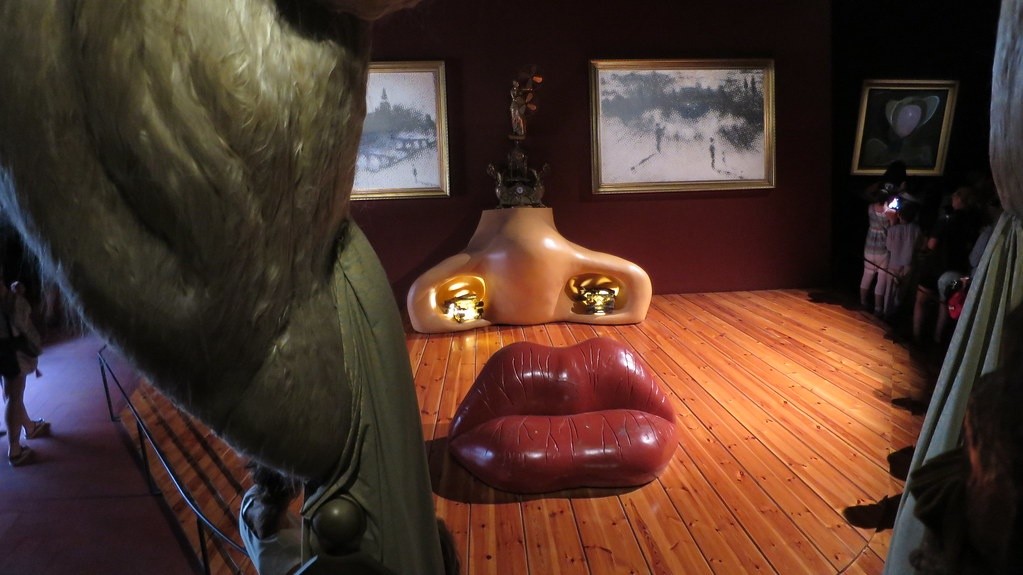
25, 417, 45, 439
9, 444, 31, 466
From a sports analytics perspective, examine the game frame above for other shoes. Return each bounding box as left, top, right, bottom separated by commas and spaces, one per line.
888, 445, 915, 467
844, 495, 888, 534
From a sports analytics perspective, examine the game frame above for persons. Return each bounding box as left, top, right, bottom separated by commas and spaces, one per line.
909, 303, 1023, 575
653, 119, 718, 172
859, 170, 1023, 346
510, 79, 532, 135
0, 262, 78, 467
238, 458, 311, 575
437, 518, 461, 575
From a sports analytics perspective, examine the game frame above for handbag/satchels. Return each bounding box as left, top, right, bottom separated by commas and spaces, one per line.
16, 333, 41, 378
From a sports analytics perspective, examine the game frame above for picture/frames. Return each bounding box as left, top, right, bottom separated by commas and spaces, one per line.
349, 58, 451, 201
588, 57, 775, 193
851, 78, 961, 177
